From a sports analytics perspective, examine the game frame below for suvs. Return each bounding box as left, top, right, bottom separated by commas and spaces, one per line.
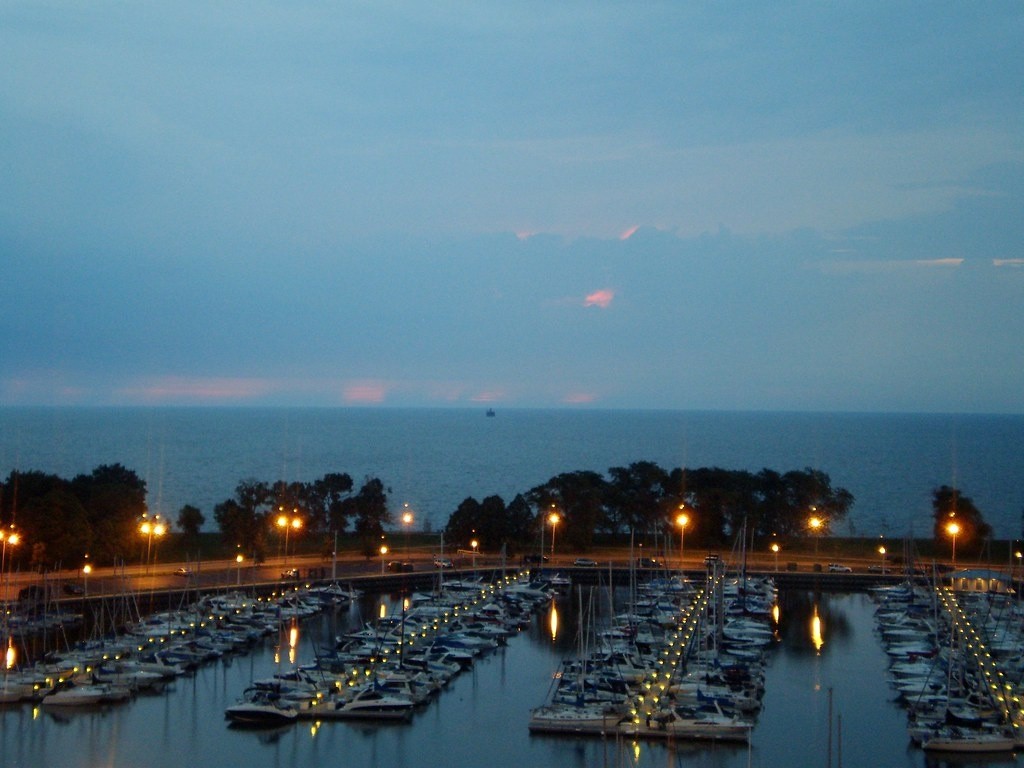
932, 563, 956, 574
638, 557, 663, 568
18, 583, 49, 602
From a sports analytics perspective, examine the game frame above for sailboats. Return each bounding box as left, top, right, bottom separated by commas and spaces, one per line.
1, 536, 1024, 768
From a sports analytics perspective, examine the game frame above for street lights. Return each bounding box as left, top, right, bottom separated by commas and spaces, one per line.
949, 523, 959, 562
677, 514, 689, 561
0, 527, 20, 579
403, 512, 413, 558
143, 522, 164, 573
548, 512, 558, 557
280, 516, 303, 564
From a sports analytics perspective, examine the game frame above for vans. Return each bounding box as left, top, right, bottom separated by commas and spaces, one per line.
574, 557, 597, 566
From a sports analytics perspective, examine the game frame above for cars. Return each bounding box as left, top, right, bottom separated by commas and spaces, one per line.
524, 553, 549, 564
433, 558, 453, 569
868, 565, 891, 574
900, 567, 925, 575
173, 567, 193, 577
62, 583, 85, 596
703, 553, 720, 567
387, 560, 414, 573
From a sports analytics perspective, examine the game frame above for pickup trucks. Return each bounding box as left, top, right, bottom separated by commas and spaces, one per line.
828, 563, 852, 573
